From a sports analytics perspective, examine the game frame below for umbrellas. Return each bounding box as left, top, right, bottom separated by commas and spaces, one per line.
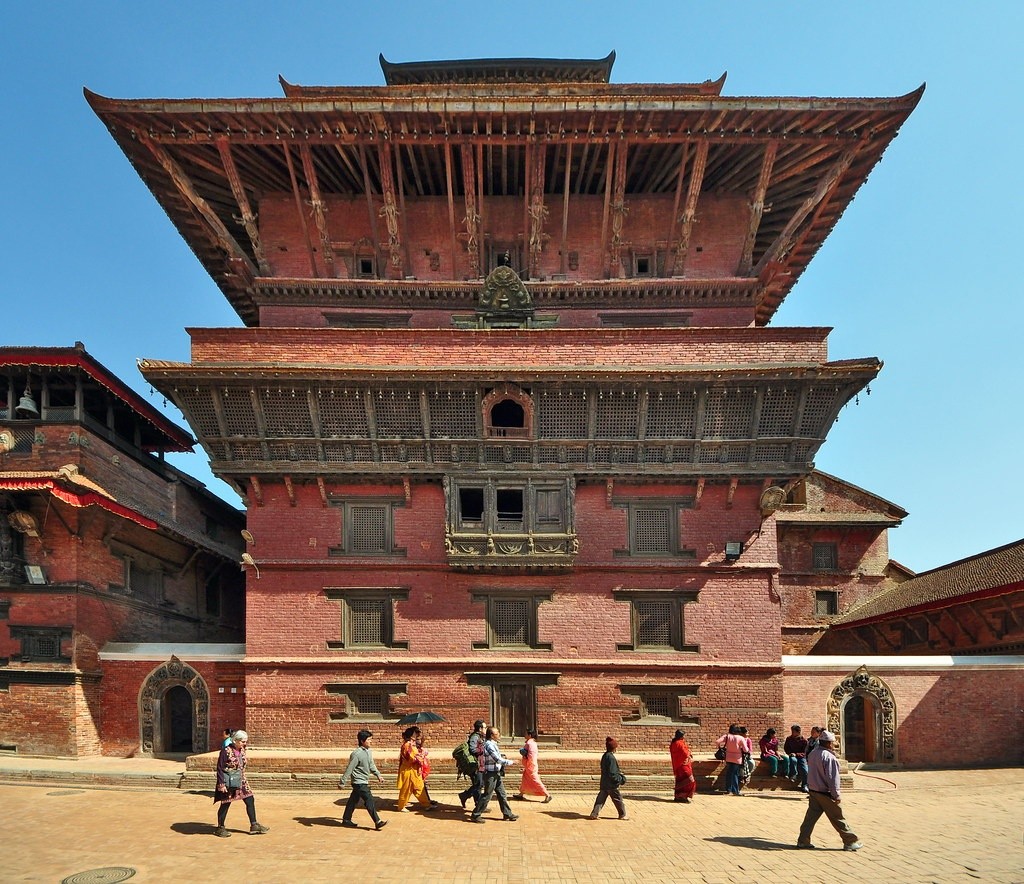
394, 711, 447, 736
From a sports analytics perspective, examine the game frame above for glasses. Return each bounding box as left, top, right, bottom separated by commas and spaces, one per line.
483, 726, 488, 729
238, 739, 248, 743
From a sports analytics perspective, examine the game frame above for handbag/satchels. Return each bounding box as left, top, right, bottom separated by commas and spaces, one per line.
453, 732, 482, 779
715, 748, 726, 760
224, 770, 241, 788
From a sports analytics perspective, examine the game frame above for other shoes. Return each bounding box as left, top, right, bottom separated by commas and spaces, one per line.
772, 775, 809, 793
470, 817, 485, 823
375, 821, 387, 829
541, 796, 552, 803
431, 800, 437, 805
844, 841, 863, 850
798, 842, 814, 848
619, 817, 630, 820
591, 816, 602, 820
503, 814, 519, 820
675, 798, 688, 802
425, 805, 438, 811
514, 795, 523, 800
739, 777, 750, 784
342, 820, 357, 827
398, 807, 409, 812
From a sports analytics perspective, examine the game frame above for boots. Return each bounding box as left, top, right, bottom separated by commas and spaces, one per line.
459, 790, 471, 808
482, 808, 492, 813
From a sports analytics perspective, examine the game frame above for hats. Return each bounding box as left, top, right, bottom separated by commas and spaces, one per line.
819, 730, 835, 742
606, 737, 617, 751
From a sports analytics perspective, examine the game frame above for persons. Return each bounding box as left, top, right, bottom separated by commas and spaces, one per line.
338, 730, 390, 829
797, 730, 863, 851
590, 737, 630, 820
512, 728, 552, 804
669, 729, 696, 803
396, 726, 439, 813
213, 728, 270, 837
715, 723, 756, 796
458, 719, 520, 824
758, 724, 826, 793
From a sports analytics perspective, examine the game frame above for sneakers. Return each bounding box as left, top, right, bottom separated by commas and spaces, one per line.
215, 826, 231, 837
250, 823, 270, 835
